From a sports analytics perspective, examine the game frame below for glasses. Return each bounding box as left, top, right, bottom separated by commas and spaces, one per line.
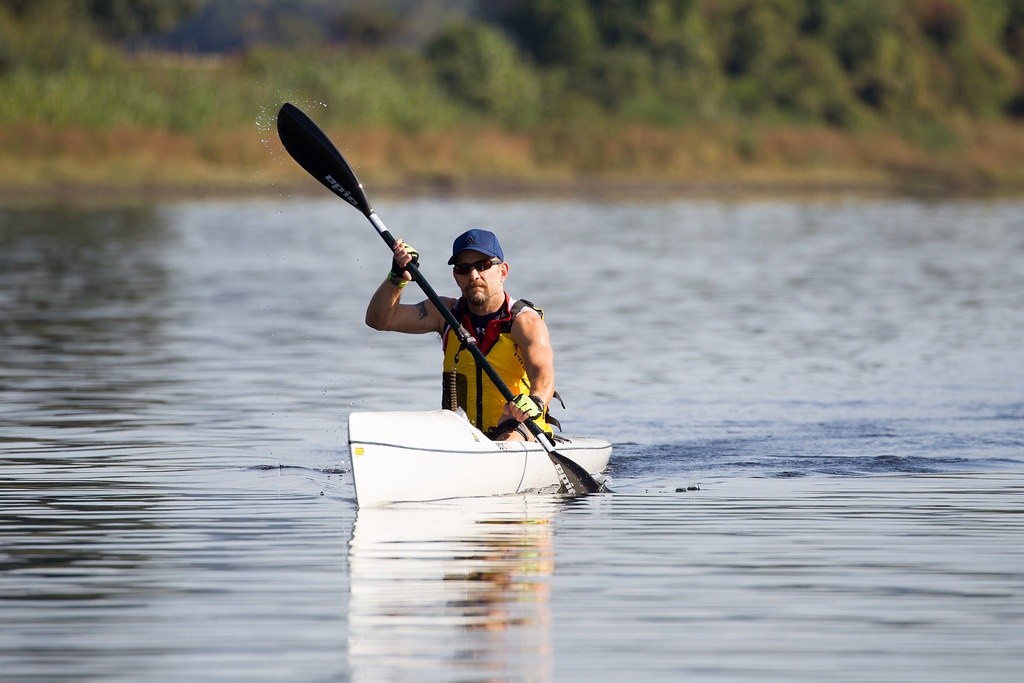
453, 259, 503, 274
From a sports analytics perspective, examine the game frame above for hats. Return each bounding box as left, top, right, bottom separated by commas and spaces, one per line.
448, 229, 504, 265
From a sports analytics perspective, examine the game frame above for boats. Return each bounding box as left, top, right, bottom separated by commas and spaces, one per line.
345, 409, 613, 508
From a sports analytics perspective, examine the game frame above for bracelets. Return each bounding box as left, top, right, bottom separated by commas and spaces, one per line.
528, 394, 546, 412
387, 271, 409, 289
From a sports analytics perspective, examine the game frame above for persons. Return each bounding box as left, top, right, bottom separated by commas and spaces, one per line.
363, 227, 556, 444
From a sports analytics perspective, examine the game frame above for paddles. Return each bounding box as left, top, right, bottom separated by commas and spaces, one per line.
277, 102, 613, 496
